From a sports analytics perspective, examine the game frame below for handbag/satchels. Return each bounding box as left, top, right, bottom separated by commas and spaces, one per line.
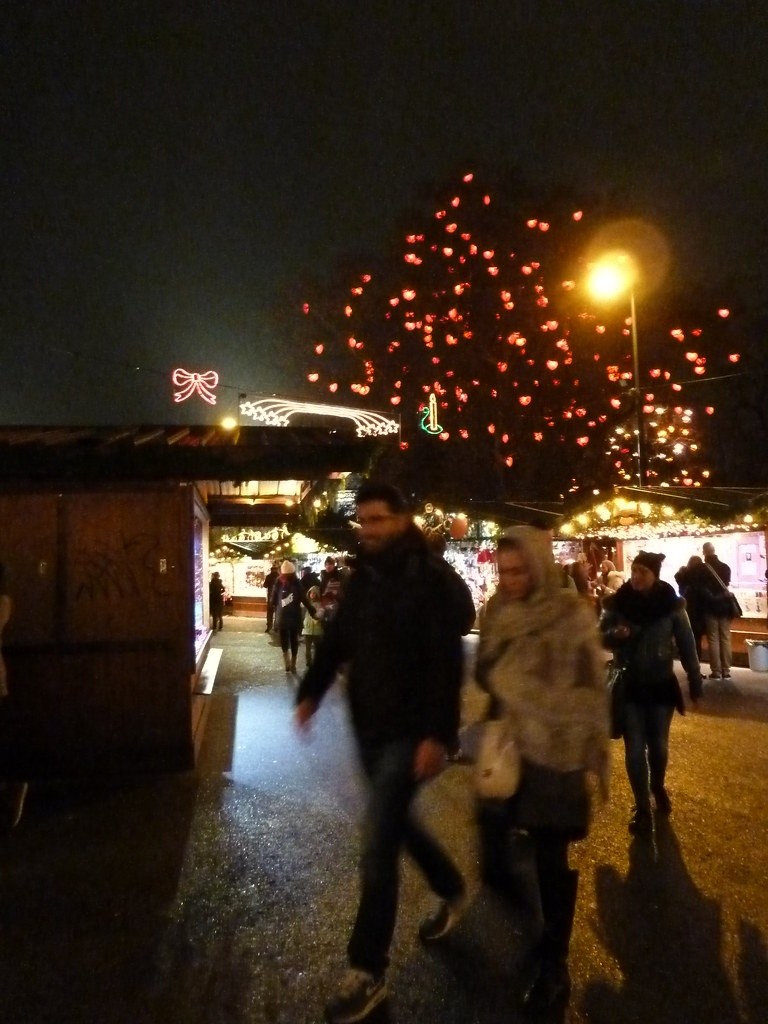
728, 594, 742, 618
605, 661, 633, 740
459, 721, 520, 801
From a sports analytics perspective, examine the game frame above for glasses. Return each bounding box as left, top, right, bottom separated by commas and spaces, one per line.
355, 516, 396, 527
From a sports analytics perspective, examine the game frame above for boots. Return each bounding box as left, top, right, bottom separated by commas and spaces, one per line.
290, 656, 296, 674
516, 869, 579, 1016
265, 621, 272, 632
305, 654, 312, 665
477, 810, 528, 901
283, 653, 291, 672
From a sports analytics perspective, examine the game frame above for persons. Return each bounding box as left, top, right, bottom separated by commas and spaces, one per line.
209, 573, 224, 630
263, 474, 743, 1024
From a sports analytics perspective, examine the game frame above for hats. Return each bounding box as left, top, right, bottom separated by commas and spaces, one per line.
632, 551, 665, 577
281, 560, 295, 574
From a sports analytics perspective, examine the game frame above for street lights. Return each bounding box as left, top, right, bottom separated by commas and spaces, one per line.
593, 259, 649, 487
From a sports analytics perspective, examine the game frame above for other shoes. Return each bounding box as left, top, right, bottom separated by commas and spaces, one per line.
655, 796, 671, 823
2, 779, 29, 828
709, 674, 721, 679
628, 801, 655, 833
699, 673, 707, 679
723, 674, 731, 678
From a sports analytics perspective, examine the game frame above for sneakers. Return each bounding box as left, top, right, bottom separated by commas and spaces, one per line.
324, 967, 387, 1024
419, 886, 467, 939
